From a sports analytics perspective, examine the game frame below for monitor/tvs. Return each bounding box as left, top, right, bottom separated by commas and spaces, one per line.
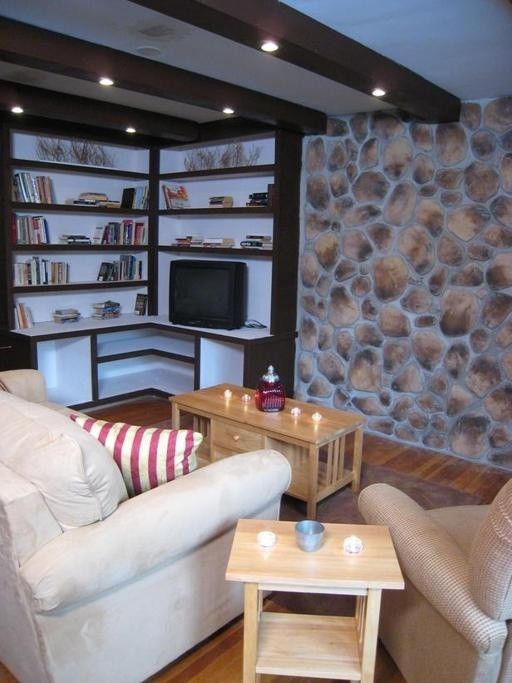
169, 259, 247, 330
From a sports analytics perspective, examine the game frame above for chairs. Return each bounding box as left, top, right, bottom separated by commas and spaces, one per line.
355, 471, 512, 683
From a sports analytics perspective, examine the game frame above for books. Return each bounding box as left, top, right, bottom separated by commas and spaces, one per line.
162, 181, 273, 250
14, 169, 147, 331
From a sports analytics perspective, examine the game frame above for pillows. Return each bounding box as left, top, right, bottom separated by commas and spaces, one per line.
66, 406, 204, 500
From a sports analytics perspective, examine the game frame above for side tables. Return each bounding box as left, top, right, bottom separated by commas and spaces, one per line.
223, 518, 405, 683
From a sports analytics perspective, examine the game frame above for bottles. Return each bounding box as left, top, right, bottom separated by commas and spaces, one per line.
255, 365, 286, 412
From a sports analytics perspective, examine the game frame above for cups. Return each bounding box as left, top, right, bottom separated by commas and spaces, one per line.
295, 519, 325, 552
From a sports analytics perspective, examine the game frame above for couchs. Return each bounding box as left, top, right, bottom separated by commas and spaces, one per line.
0, 367, 294, 683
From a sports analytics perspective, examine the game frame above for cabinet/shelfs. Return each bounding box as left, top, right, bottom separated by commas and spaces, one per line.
0, 123, 305, 410
